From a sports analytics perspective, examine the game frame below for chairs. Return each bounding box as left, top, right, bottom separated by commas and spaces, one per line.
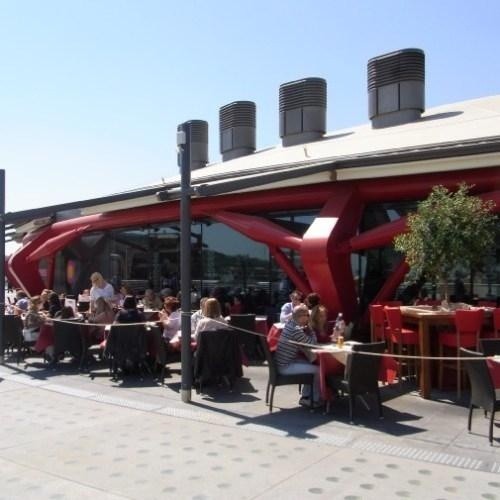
326, 299, 499, 448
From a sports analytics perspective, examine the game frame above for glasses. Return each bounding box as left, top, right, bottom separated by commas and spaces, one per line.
299, 314, 309, 317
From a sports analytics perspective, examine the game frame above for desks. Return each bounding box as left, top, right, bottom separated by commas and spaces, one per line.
308, 341, 390, 402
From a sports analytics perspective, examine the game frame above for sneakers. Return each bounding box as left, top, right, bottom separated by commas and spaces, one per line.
299, 395, 313, 408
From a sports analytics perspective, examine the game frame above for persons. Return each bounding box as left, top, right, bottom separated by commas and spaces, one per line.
5, 267, 327, 359
273, 303, 321, 412
109, 253, 469, 314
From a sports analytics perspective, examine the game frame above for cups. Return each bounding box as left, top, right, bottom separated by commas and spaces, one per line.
336, 336, 344, 348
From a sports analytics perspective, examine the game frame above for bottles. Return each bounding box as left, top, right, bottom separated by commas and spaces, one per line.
335, 312, 345, 346
